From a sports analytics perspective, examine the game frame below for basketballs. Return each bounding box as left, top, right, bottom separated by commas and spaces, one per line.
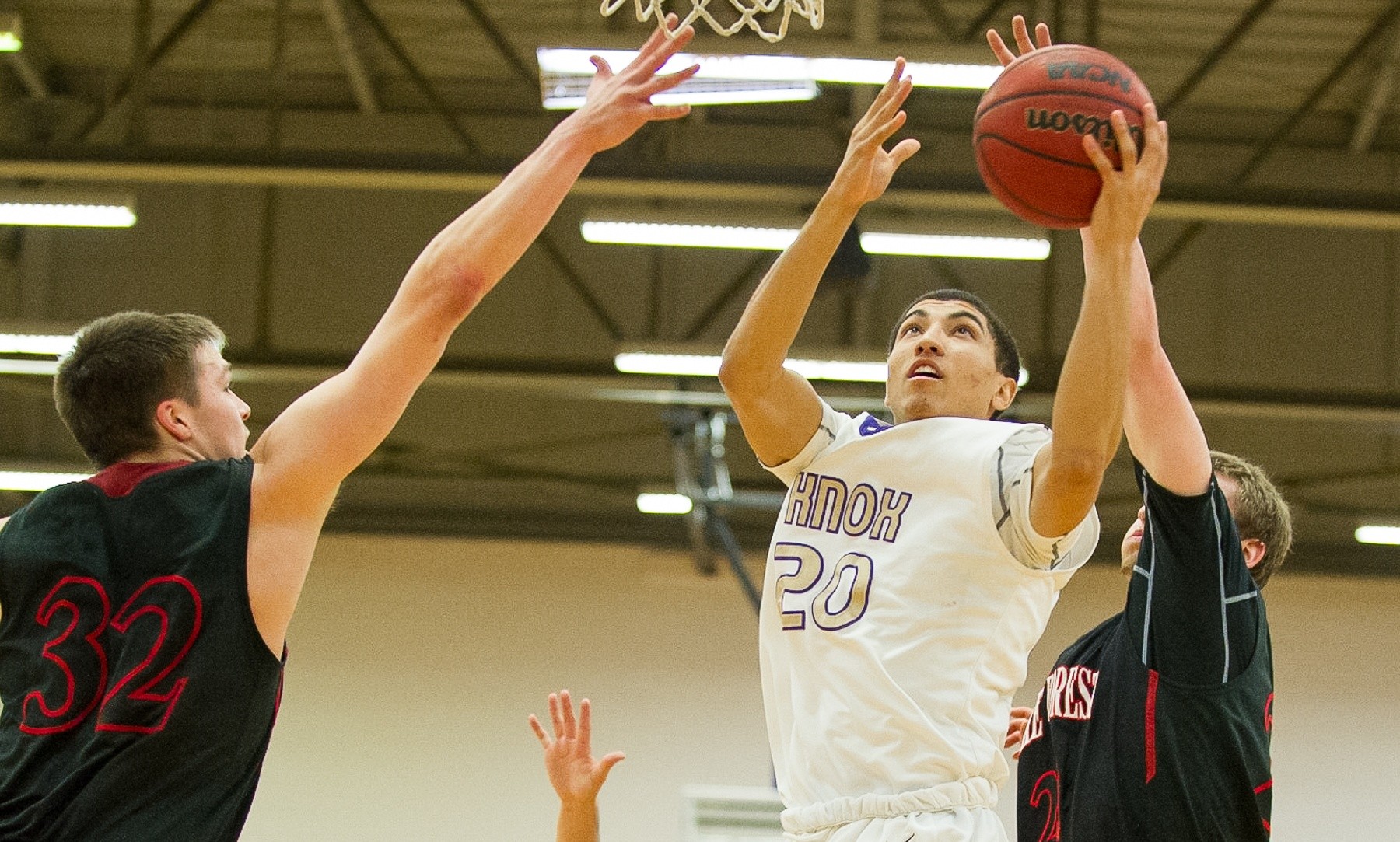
972, 43, 1157, 229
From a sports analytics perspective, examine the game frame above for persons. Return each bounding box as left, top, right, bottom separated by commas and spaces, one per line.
718, 56, 1169, 842
530, 690, 624, 842
0, 11, 700, 841
985, 16, 1292, 842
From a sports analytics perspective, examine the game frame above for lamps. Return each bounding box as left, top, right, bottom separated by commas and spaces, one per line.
0, 181, 139, 494
536, 44, 1052, 513
1353, 520, 1400, 549
